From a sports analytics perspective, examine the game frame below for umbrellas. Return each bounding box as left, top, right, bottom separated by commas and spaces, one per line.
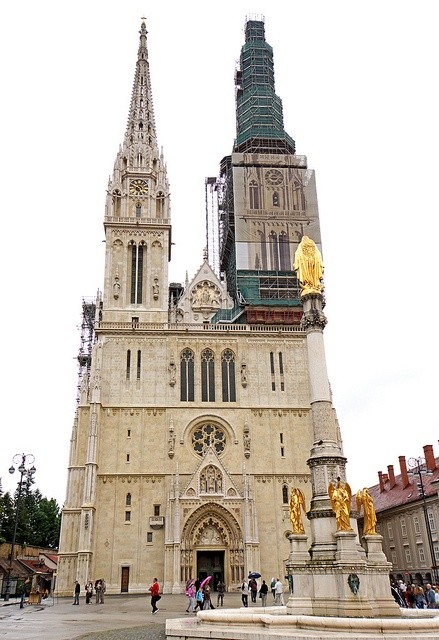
248, 573, 261, 578
200, 576, 212, 588
186, 578, 195, 591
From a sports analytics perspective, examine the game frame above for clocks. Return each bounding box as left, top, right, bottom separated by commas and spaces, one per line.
128, 179, 148, 195
264, 169, 284, 185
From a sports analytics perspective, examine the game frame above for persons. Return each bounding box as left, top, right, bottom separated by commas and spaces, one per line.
204, 580, 215, 609
271, 578, 277, 599
100, 578, 106, 604
42, 586, 49, 599
331, 481, 355, 532
72, 581, 80, 605
259, 580, 268, 606
391, 579, 439, 609
20, 579, 29, 608
272, 578, 284, 606
195, 587, 203, 610
289, 487, 305, 534
147, 578, 161, 614
241, 580, 249, 607
249, 578, 257, 603
203, 588, 211, 610
217, 579, 226, 607
362, 488, 377, 535
85, 580, 93, 605
186, 584, 197, 612
94, 579, 102, 603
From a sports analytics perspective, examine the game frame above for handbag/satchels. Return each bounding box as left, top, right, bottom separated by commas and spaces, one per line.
153, 596, 161, 601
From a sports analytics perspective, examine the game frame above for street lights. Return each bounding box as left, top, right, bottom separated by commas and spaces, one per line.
407, 457, 439, 588
4, 453, 36, 602
